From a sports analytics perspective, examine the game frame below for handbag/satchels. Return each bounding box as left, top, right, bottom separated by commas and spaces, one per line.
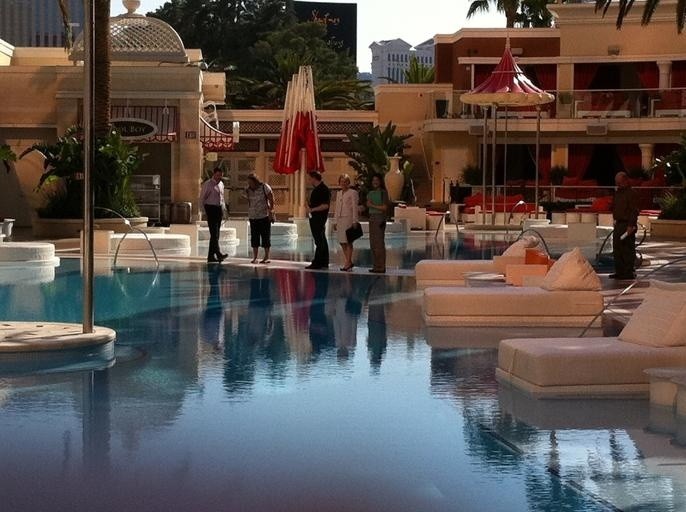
345, 222, 363, 243
268, 215, 276, 223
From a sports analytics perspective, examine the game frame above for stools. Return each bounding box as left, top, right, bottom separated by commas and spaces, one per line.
479, 209, 659, 230
426, 210, 447, 230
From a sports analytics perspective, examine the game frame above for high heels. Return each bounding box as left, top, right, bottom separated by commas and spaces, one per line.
340, 263, 354, 271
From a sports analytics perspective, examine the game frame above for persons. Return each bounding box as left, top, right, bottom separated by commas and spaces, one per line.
241, 173, 275, 263
305, 171, 331, 269
609, 172, 640, 280
200, 168, 228, 262
367, 174, 388, 273
334, 174, 363, 272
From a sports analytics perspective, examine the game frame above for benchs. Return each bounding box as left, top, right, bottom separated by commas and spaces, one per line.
578, 93, 632, 118
655, 90, 686, 118
461, 193, 536, 222
498, 106, 552, 119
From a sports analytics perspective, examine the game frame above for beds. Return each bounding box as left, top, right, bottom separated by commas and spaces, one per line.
415, 237, 685, 400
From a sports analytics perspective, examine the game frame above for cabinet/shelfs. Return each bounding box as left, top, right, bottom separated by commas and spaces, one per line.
128, 174, 160, 227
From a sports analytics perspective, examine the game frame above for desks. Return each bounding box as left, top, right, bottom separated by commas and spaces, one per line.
394, 206, 426, 231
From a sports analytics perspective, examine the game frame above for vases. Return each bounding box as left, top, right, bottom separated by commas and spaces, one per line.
384, 157, 404, 202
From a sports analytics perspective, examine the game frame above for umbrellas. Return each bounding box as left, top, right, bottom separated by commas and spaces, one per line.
273, 65, 326, 218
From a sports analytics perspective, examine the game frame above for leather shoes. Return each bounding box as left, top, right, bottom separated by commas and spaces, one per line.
251, 261, 259, 263
615, 275, 636, 280
217, 254, 229, 262
260, 261, 271, 263
207, 258, 224, 262
312, 264, 329, 268
305, 266, 322, 270
609, 273, 635, 278
